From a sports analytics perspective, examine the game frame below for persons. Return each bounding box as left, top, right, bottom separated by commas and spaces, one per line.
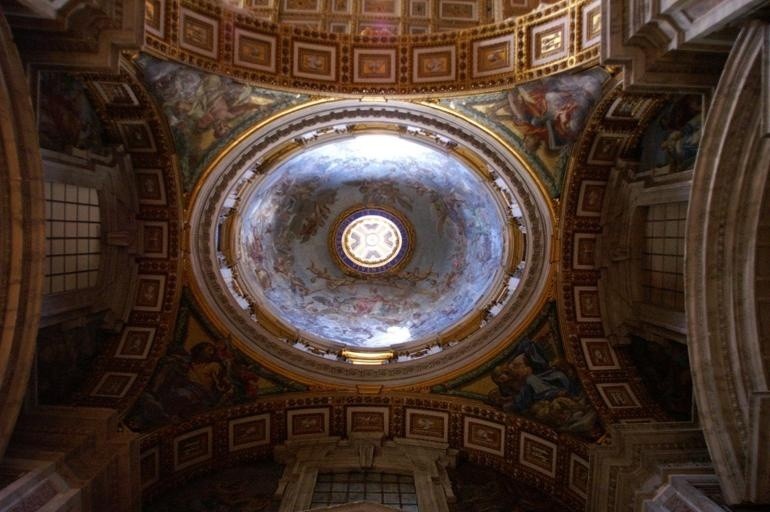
152, 342, 226, 405
659, 94, 703, 173
173, 75, 269, 138
491, 351, 568, 412
508, 93, 549, 155
